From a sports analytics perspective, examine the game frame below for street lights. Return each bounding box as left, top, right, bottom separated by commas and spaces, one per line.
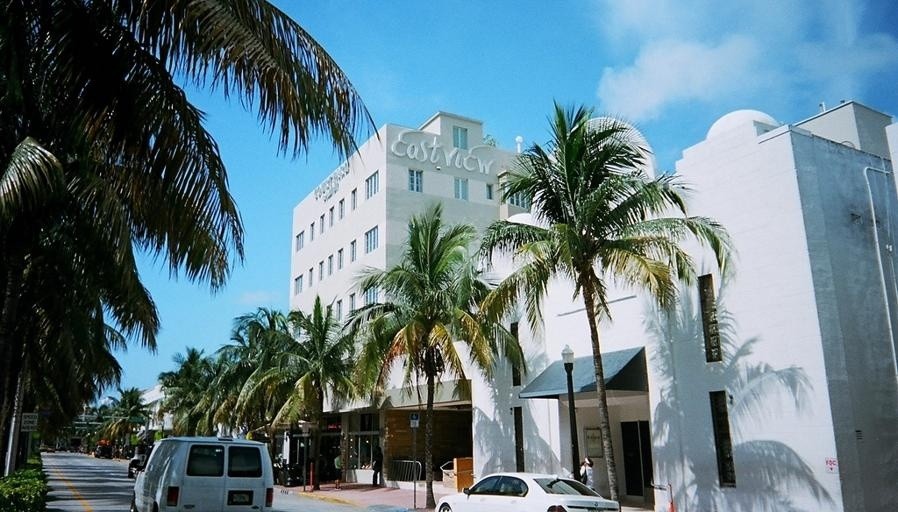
562, 343, 582, 482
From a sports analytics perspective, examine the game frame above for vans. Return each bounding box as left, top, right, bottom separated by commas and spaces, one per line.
130, 436, 275, 511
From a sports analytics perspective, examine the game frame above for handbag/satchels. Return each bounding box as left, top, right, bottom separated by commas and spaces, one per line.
579, 468, 588, 485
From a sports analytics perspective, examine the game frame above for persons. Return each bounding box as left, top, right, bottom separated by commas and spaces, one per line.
333, 452, 344, 490
578, 455, 595, 491
372, 446, 382, 486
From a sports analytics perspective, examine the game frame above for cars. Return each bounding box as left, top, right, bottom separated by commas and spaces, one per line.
434, 473, 620, 512
39, 444, 112, 458
